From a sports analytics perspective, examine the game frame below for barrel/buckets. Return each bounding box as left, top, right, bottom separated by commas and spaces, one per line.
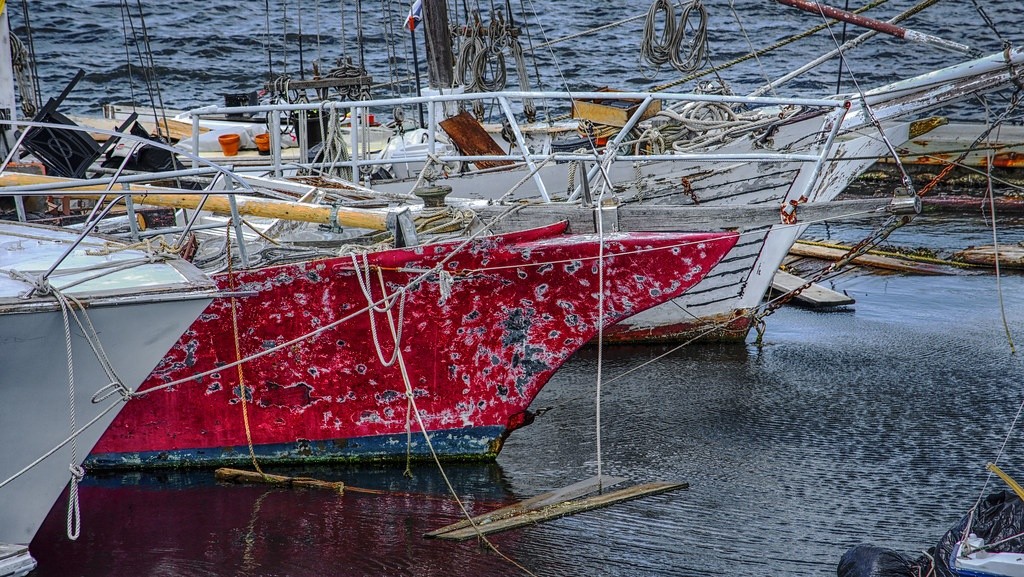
292, 111, 330, 151
218, 134, 239, 156
255, 134, 270, 153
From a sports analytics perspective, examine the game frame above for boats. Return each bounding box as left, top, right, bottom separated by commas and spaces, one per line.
837, 474, 1023, 576
12, 48, 742, 470
8, 0, 1024, 341
0, 222, 227, 577
